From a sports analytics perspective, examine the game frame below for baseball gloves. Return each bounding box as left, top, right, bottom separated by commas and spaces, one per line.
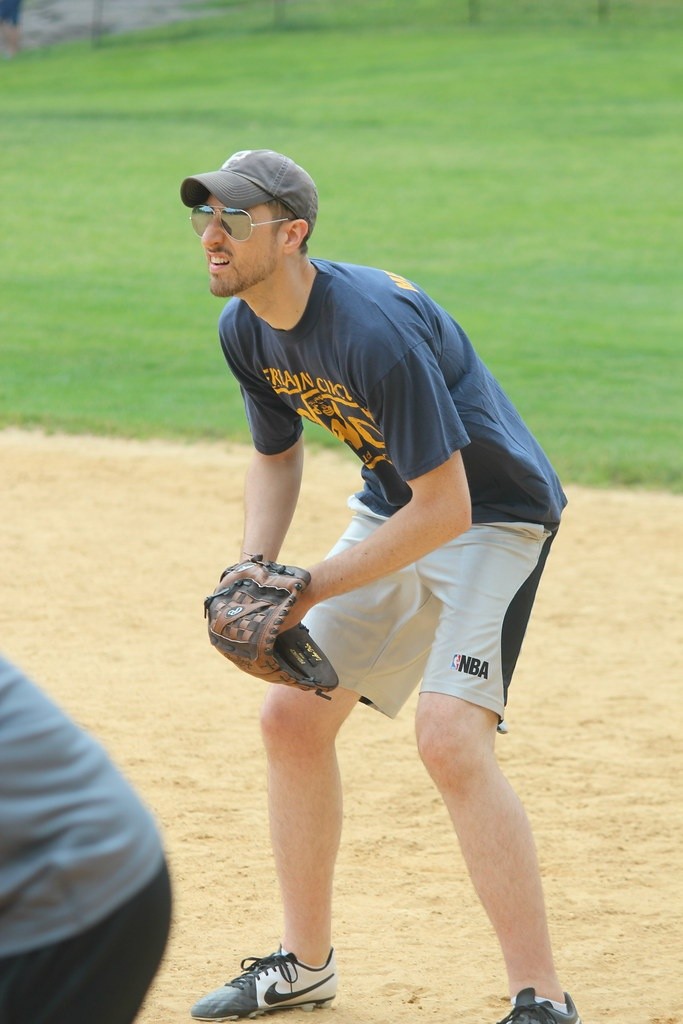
204, 552, 340, 701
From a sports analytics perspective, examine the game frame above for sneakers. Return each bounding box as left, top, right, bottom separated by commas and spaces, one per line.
495, 987, 582, 1024
191, 943, 336, 1021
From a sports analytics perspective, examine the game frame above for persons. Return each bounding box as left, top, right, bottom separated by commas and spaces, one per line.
180, 150, 584, 1024
0, 655, 174, 1024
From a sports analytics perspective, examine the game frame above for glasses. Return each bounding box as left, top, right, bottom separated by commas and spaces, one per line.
189, 204, 289, 240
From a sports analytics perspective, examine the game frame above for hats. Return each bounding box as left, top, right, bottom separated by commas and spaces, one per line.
180, 149, 318, 238
